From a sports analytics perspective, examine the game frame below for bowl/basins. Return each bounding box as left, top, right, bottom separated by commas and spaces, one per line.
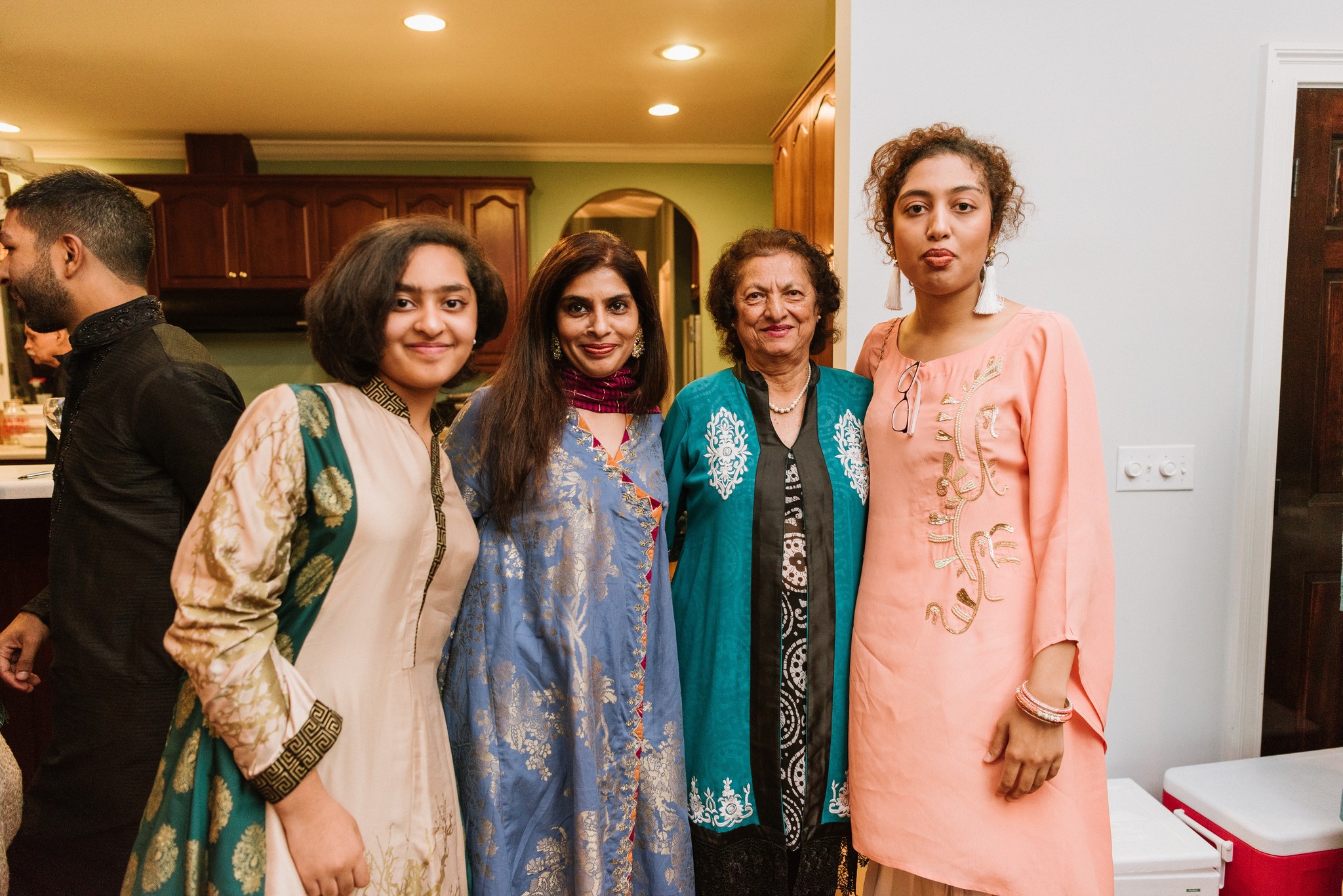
3, 404, 46, 448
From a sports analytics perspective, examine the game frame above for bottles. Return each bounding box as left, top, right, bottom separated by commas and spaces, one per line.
0, 399, 29, 445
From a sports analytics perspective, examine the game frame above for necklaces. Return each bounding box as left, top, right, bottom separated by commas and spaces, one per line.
768, 363, 812, 415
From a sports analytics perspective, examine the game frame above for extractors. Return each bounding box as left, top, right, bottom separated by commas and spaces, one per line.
160, 286, 308, 331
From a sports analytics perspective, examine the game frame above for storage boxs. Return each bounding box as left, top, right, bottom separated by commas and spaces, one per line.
1107, 777, 1222, 896
1162, 743, 1343, 896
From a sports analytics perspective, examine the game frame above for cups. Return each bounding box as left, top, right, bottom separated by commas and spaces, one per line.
43, 398, 65, 440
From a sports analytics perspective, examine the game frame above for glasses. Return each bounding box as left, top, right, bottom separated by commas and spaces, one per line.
892, 361, 925, 434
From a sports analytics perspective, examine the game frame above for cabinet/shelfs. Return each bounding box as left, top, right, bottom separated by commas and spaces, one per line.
111, 174, 535, 373
767, 44, 835, 367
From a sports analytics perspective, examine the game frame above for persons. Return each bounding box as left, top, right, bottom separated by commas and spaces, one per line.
849, 125, 1116, 896
437, 231, 695, 896
0, 168, 245, 896
23, 320, 73, 464
660, 229, 874, 896
124, 218, 510, 896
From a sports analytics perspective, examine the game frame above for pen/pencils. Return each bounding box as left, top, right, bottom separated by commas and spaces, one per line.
18, 471, 53, 480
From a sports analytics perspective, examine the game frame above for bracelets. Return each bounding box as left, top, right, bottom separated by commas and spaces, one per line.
1014, 681, 1073, 725
